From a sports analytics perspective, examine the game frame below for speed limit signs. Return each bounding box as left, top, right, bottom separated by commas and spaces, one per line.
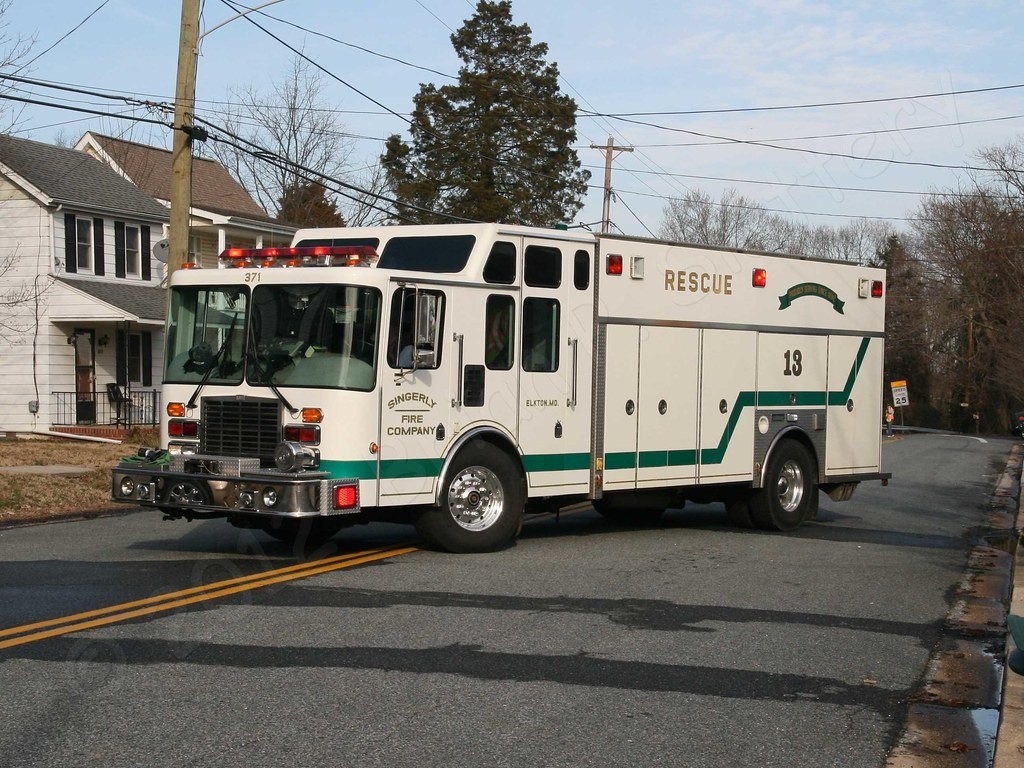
890, 380, 910, 408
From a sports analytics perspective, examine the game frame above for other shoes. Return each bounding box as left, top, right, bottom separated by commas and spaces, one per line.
888, 434, 894, 438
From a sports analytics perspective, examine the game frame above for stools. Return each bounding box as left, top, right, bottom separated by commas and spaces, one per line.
106, 382, 133, 426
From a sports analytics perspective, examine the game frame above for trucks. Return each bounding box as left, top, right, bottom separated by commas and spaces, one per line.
108, 221, 894, 554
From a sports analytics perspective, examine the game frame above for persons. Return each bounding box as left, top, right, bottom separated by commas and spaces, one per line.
885, 404, 895, 435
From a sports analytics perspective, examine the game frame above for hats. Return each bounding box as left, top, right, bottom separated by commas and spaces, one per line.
887, 404, 891, 406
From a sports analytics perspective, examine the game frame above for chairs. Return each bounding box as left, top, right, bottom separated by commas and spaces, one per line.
289, 306, 335, 350
